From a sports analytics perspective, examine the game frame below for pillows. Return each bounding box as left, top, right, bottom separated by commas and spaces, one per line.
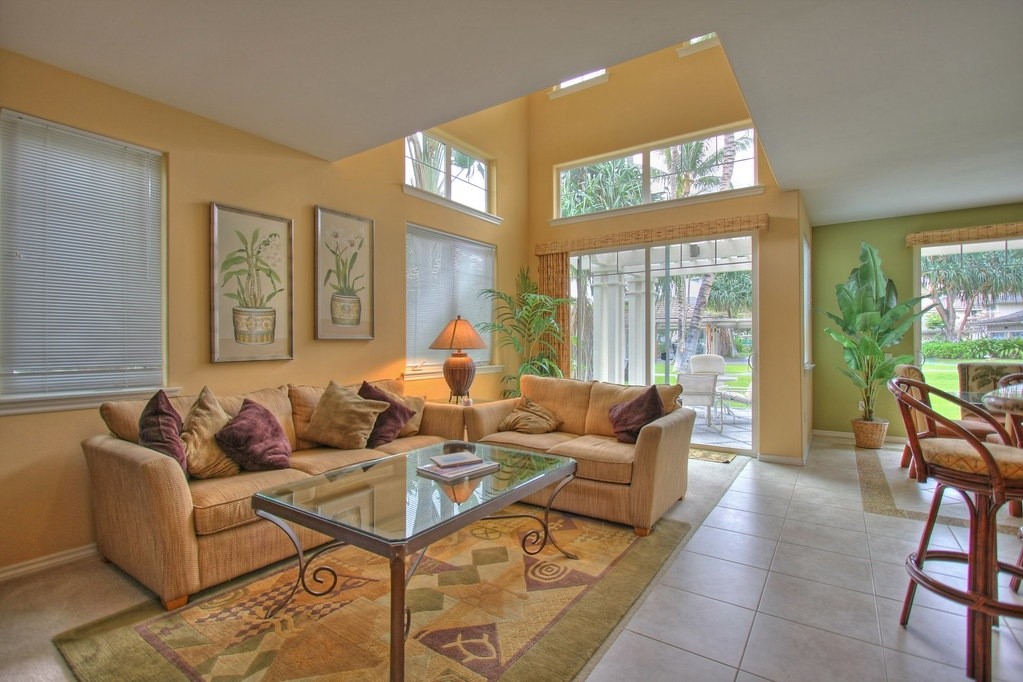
139, 389, 189, 479
214, 398, 291, 473
356, 381, 416, 449
609, 384, 666, 444
376, 385, 427, 438
181, 385, 242, 478
296, 380, 390, 449
500, 393, 564, 435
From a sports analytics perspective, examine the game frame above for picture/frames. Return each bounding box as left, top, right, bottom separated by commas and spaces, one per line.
315, 205, 375, 341
210, 200, 295, 363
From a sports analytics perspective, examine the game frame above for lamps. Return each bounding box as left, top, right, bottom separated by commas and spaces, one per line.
438, 477, 481, 506
429, 315, 488, 405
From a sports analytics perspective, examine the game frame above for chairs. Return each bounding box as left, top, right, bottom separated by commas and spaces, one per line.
894, 365, 997, 477
887, 376, 1023, 682
1000, 373, 1023, 519
677, 354, 751, 433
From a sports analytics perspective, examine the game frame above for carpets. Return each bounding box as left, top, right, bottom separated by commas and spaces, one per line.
51, 518, 690, 682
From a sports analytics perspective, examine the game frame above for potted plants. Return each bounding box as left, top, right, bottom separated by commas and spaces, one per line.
813, 240, 941, 449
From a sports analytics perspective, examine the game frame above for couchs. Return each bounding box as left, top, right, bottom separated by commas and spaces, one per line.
463, 376, 696, 536
79, 379, 465, 612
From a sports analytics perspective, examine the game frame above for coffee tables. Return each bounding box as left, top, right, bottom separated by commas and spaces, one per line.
252, 437, 580, 682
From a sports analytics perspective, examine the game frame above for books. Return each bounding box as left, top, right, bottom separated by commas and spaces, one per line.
416, 458, 500, 481
416, 468, 500, 485
429, 451, 484, 469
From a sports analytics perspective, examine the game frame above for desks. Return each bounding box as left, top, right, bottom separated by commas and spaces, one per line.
428, 396, 494, 405
716, 376, 738, 381
